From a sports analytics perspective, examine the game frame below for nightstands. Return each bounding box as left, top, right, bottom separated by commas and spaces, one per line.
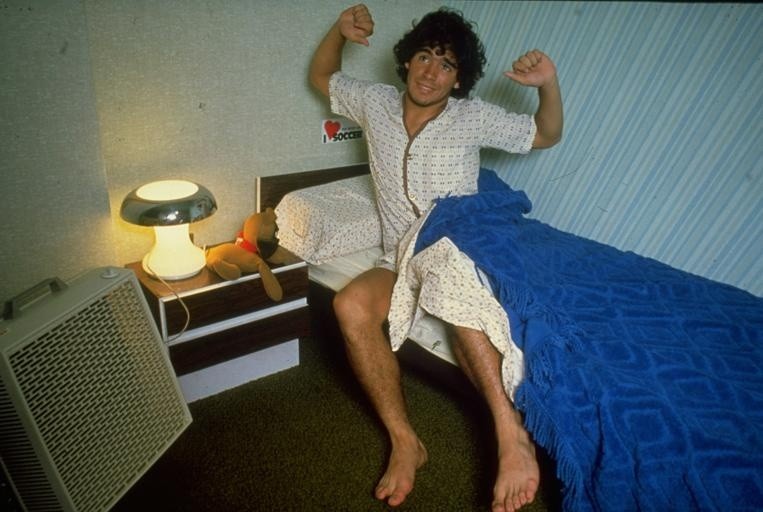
124, 238, 310, 405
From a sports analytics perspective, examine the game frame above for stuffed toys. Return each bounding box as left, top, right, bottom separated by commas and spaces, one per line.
205, 207, 287, 304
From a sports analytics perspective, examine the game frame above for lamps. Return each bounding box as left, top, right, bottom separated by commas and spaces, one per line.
119, 179, 218, 283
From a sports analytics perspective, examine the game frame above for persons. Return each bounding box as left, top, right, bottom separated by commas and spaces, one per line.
308, 3, 567, 512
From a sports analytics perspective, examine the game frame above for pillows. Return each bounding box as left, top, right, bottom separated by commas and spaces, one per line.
274, 172, 383, 267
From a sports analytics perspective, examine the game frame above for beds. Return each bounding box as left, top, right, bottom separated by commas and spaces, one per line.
254, 162, 761, 512
